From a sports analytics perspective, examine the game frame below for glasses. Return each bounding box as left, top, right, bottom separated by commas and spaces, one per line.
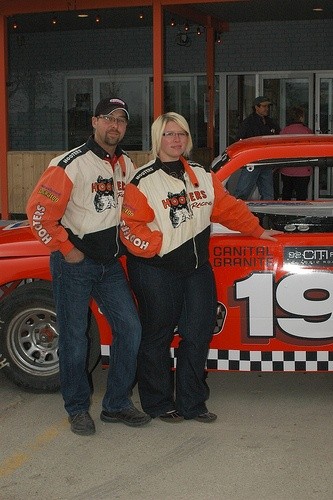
163, 131, 187, 138
96, 115, 128, 126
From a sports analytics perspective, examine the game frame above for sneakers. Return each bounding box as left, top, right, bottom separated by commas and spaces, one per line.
100, 405, 152, 427
68, 412, 96, 435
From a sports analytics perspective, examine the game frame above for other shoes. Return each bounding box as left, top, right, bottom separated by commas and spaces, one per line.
158, 409, 185, 423
193, 411, 217, 423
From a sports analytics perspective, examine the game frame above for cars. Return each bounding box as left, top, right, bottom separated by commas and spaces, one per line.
0, 133, 333, 394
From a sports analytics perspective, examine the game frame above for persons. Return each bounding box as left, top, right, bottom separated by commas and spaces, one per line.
119, 112, 282, 425
276, 106, 314, 201
233, 95, 279, 202
26, 98, 151, 435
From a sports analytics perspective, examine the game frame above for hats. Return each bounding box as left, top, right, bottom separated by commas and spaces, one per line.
252, 96, 273, 106
95, 97, 130, 120
